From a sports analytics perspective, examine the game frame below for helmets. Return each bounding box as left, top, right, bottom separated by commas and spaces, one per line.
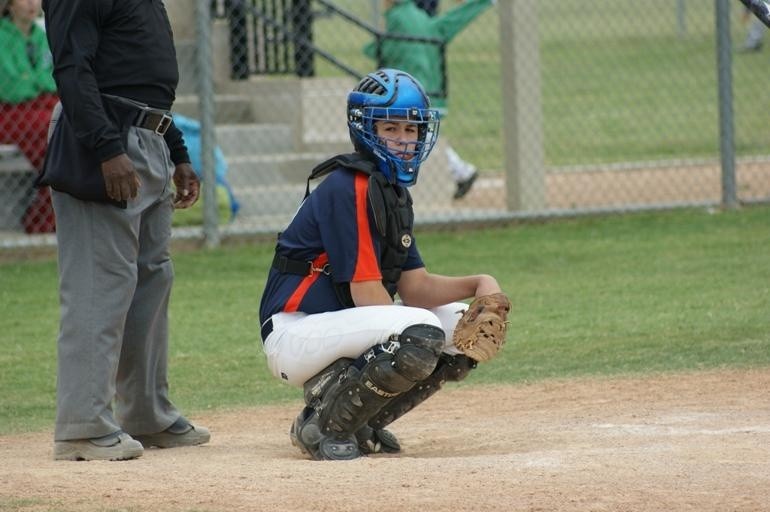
347, 67, 439, 188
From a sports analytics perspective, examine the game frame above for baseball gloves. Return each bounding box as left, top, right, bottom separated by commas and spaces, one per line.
452, 292, 512, 361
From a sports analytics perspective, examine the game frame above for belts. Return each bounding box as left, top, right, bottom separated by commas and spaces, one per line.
131, 110, 172, 136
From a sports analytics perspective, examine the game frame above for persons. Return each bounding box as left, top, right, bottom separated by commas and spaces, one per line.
0, 0, 63, 235
259, 68, 509, 462
41, 0, 210, 461
366, 0, 478, 200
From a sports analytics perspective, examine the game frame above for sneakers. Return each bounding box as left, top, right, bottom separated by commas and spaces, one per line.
130, 415, 210, 448
454, 162, 478, 199
289, 405, 329, 460
354, 422, 399, 454
52, 430, 144, 461
